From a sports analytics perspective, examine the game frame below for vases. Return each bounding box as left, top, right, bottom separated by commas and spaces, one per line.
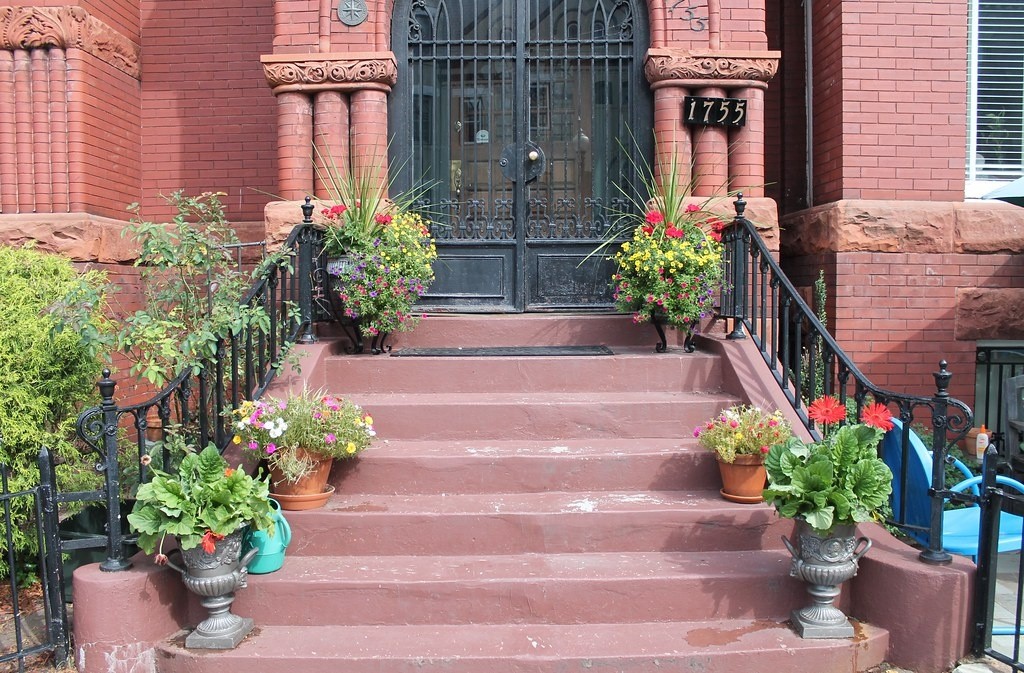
314, 268, 395, 354
781, 517, 871, 638
264, 445, 335, 510
164, 516, 259, 648
965, 427, 993, 454
716, 448, 770, 503
638, 302, 705, 354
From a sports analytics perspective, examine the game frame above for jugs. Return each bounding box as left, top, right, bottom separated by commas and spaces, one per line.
246, 498, 292, 574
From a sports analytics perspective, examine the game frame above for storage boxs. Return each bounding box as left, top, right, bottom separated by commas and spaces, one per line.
60, 494, 141, 601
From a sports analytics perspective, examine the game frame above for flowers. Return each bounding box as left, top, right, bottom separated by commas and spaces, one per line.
610, 204, 727, 330
693, 400, 793, 462
321, 204, 438, 337
128, 441, 279, 565
763, 395, 894, 527
219, 383, 389, 487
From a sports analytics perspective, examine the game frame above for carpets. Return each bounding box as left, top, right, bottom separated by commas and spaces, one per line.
390, 344, 613, 356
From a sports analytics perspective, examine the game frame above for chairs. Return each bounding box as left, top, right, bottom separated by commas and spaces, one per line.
882, 416, 1024, 636
1002, 375, 1024, 493
